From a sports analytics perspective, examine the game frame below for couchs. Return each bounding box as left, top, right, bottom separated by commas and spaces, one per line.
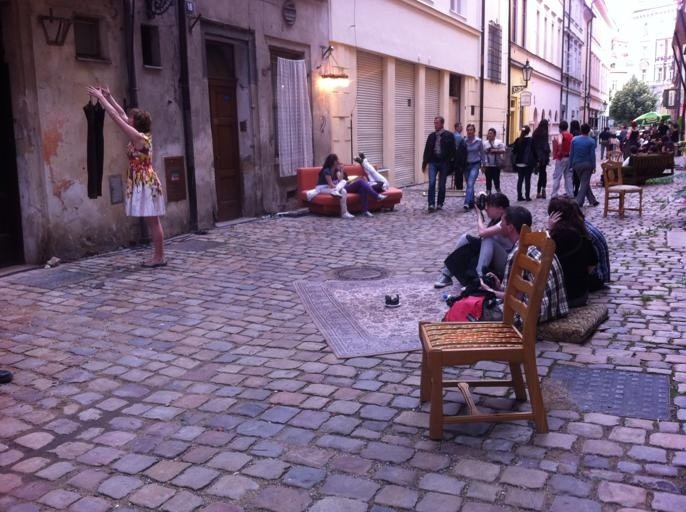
296, 163, 403, 216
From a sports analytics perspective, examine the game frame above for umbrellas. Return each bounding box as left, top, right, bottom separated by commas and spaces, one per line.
632, 110, 671, 125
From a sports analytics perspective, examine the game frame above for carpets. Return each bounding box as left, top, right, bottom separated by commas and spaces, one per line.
293, 271, 466, 359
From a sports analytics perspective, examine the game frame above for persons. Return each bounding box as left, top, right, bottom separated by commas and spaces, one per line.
622, 145, 638, 167
332, 162, 388, 219
479, 206, 570, 333
421, 117, 456, 212
588, 129, 597, 147
552, 120, 575, 199
85, 83, 167, 267
352, 152, 388, 193
453, 123, 465, 190
570, 120, 581, 196
622, 130, 640, 160
615, 127, 622, 139
569, 124, 599, 208
480, 128, 506, 195
316, 154, 355, 219
462, 124, 486, 209
599, 127, 611, 160
626, 121, 639, 140
574, 201, 611, 292
434, 192, 515, 292
511, 126, 536, 202
637, 119, 679, 153
619, 126, 628, 151
532, 119, 551, 198
546, 196, 591, 307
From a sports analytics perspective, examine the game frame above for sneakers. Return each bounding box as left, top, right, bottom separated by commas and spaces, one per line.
437, 205, 443, 210
330, 190, 344, 198
433, 281, 454, 288
428, 205, 435, 213
342, 214, 355, 220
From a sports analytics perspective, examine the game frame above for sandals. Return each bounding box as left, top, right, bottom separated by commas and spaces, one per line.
364, 211, 373, 219
377, 195, 390, 202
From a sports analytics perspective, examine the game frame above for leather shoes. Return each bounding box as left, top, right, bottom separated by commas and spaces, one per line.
518, 197, 524, 201
358, 150, 365, 159
142, 260, 168, 267
354, 156, 362, 163
463, 205, 470, 210
594, 202, 600, 207
141, 261, 163, 268
543, 193, 546, 199
526, 197, 533, 201
536, 195, 541, 199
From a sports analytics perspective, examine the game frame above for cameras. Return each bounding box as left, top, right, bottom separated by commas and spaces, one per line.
443, 275, 504, 310
470, 193, 486, 210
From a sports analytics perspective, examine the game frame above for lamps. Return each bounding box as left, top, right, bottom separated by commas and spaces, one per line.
512, 59, 533, 94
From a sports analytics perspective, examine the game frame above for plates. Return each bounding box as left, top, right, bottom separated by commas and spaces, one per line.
384, 302, 402, 308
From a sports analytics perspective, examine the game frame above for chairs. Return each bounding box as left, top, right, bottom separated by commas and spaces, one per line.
607, 150, 623, 162
600, 159, 643, 219
418, 223, 556, 441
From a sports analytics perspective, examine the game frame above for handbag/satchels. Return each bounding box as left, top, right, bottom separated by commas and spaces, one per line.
488, 138, 505, 170
300, 188, 320, 201
